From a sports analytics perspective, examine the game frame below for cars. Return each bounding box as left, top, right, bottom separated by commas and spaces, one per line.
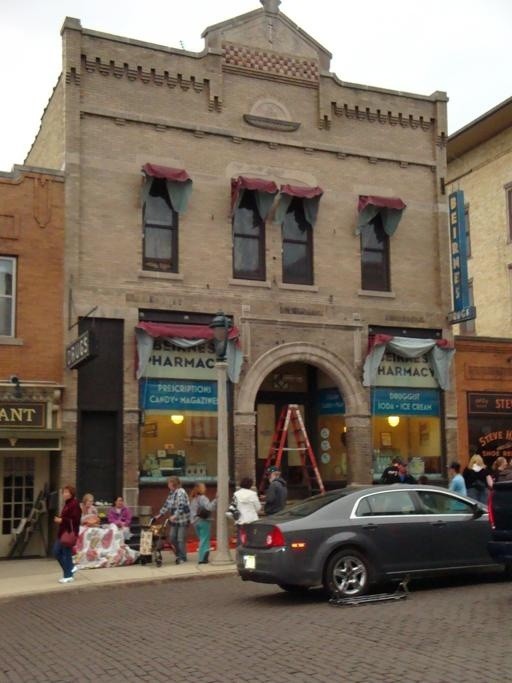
233, 480, 508, 601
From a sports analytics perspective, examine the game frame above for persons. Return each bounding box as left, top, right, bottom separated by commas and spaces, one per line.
105, 495, 132, 527
258, 464, 288, 515
380, 451, 511, 503
50, 484, 82, 583
232, 477, 262, 550
154, 475, 188, 565
78, 493, 101, 528
188, 479, 218, 564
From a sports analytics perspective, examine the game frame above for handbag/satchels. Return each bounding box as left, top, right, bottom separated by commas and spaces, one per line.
60, 531, 76, 548
224, 501, 236, 521
196, 506, 210, 519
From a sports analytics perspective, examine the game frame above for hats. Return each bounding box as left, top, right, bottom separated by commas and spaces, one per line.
446, 462, 461, 468
400, 460, 407, 466
391, 458, 400, 463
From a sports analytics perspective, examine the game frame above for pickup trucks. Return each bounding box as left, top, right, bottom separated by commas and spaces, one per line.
487, 479, 512, 566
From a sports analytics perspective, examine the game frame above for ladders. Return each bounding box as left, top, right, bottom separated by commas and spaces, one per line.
257, 404, 325, 502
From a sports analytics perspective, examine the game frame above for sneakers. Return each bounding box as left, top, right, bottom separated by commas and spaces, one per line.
175, 557, 187, 564
198, 560, 209, 563
71, 566, 79, 573
59, 577, 73, 584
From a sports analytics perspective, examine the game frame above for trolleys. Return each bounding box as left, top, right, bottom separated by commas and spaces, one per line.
140, 516, 176, 566
